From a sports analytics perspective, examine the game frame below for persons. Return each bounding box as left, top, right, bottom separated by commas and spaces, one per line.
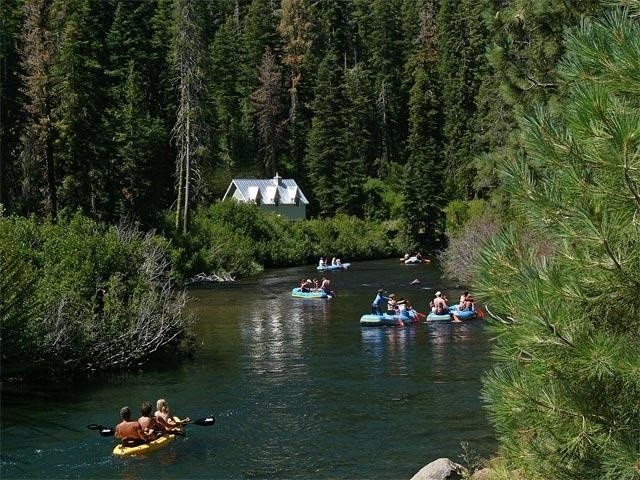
372, 289, 419, 321
416, 253, 422, 260
115, 398, 191, 439
430, 291, 448, 315
332, 257, 341, 266
301, 277, 331, 293
404, 252, 410, 260
457, 290, 473, 311
319, 257, 325, 267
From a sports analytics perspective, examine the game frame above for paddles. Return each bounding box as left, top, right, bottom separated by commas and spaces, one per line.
87, 424, 117, 430
400, 258, 405, 261
99, 428, 186, 437
422, 259, 430, 263
179, 417, 216, 426
446, 308, 461, 323
341, 265, 348, 270
122, 438, 148, 447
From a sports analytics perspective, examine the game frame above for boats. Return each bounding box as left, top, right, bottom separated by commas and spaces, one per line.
291, 286, 328, 299
404, 256, 424, 265
316, 262, 353, 274
359, 304, 420, 327
110, 415, 186, 461
425, 302, 480, 323
